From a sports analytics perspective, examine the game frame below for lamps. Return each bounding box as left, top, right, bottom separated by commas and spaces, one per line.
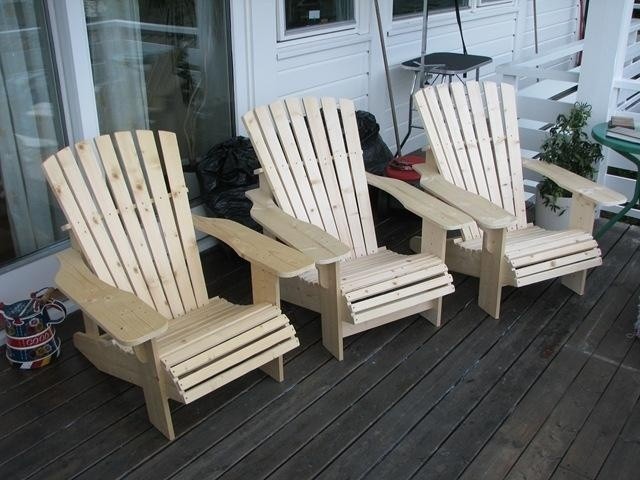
413, 77, 629, 322
37, 127, 316, 443
239, 91, 476, 366
8, 37, 204, 206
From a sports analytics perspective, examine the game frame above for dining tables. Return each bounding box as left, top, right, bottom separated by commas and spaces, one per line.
531, 102, 604, 232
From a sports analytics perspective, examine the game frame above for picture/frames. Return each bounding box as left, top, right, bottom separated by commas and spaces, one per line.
592, 119, 640, 241
393, 51, 494, 160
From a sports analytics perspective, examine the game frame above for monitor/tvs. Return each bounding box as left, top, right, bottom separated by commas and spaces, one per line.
611, 116, 635, 130
606, 126, 640, 144
